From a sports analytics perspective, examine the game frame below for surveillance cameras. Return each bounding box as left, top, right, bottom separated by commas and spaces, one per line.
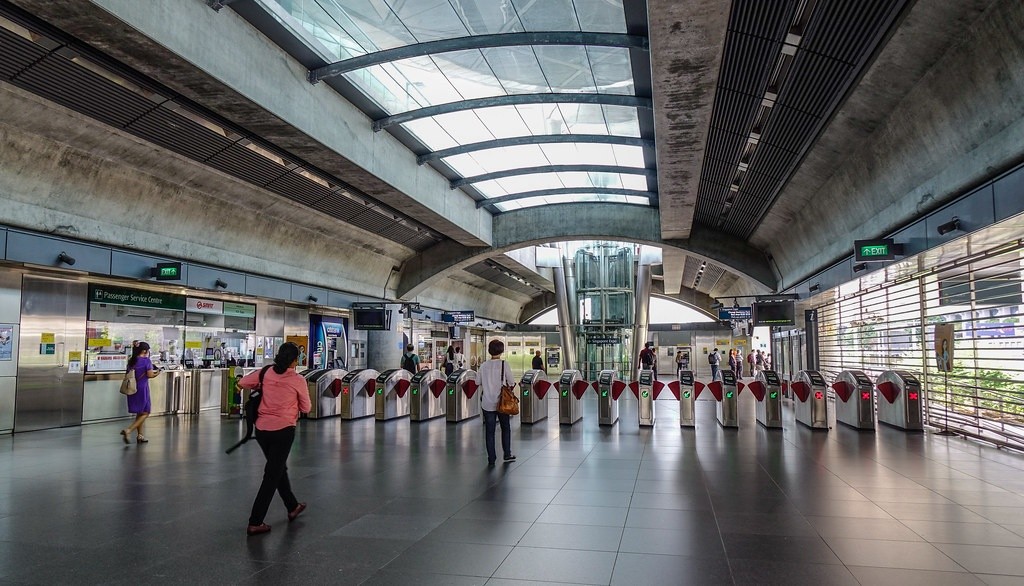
711, 303, 723, 310
412, 308, 424, 314
399, 308, 407, 314
733, 304, 739, 310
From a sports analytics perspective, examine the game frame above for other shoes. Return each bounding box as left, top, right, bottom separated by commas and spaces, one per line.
287, 502, 307, 521
137, 434, 149, 443
488, 460, 495, 464
503, 454, 517, 462
120, 428, 131, 444
247, 522, 272, 536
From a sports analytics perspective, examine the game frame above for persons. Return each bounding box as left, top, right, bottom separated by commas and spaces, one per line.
400, 343, 420, 374
475, 339, 517, 464
234, 375, 243, 403
708, 348, 722, 381
238, 342, 311, 535
747, 349, 771, 377
443, 345, 466, 377
729, 348, 744, 380
119, 342, 160, 444
638, 343, 657, 381
676, 351, 682, 375
532, 350, 545, 371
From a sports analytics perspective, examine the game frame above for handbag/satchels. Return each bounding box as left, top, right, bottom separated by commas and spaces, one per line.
439, 358, 447, 371
458, 360, 462, 367
225, 364, 274, 455
496, 384, 520, 415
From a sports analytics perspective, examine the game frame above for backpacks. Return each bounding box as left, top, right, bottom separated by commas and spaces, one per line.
403, 353, 416, 374
708, 352, 717, 364
747, 354, 754, 363
643, 351, 652, 363
119, 357, 147, 395
676, 356, 680, 364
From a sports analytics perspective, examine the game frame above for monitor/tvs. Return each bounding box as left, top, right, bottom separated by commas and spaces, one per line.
354, 309, 392, 330
751, 301, 795, 326
